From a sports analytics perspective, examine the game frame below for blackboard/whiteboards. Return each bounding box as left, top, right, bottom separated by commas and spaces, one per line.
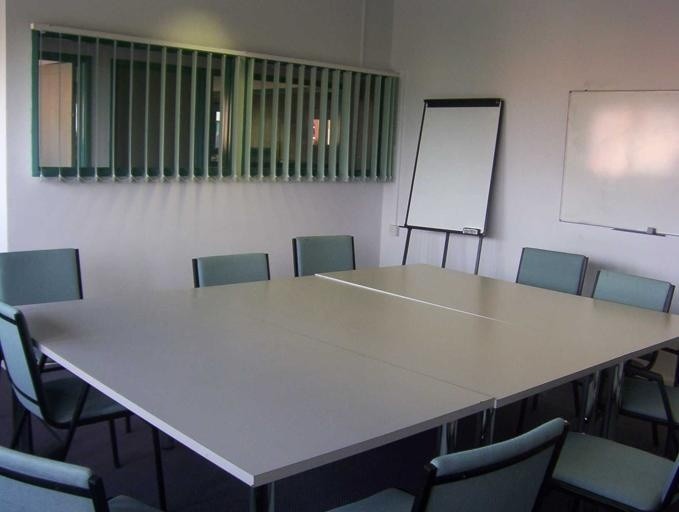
559, 89, 679, 237
405, 98, 503, 236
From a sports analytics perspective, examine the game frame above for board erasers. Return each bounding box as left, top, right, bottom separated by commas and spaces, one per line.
647, 227, 656, 235
463, 228, 480, 235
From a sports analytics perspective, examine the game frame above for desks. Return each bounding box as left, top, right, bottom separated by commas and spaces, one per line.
66, 257, 678, 476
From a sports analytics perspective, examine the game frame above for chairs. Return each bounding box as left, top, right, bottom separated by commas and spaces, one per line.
3, 442, 160, 512
1, 306, 172, 468
591, 265, 673, 411
291, 238, 364, 275
509, 243, 587, 299
334, 422, 569, 506
573, 428, 677, 511
190, 249, 273, 287
1, 244, 88, 303
